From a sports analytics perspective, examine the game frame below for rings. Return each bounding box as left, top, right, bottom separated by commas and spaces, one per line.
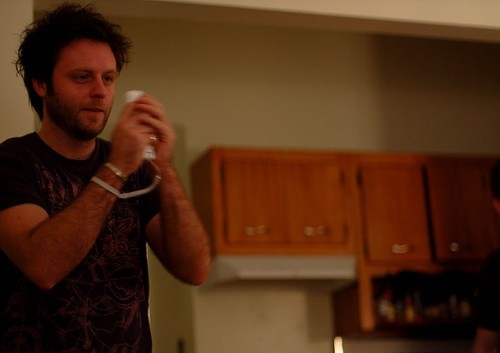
150, 134, 157, 143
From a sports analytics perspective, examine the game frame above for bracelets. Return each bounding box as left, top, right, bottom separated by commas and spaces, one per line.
104, 163, 129, 182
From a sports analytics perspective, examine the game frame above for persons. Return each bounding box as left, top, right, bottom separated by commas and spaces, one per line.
0, 4, 211, 353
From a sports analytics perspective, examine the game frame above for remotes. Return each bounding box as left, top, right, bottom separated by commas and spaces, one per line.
124, 90, 157, 159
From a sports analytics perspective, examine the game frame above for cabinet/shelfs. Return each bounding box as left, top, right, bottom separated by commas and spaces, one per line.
189, 146, 359, 257
333, 151, 499, 342
